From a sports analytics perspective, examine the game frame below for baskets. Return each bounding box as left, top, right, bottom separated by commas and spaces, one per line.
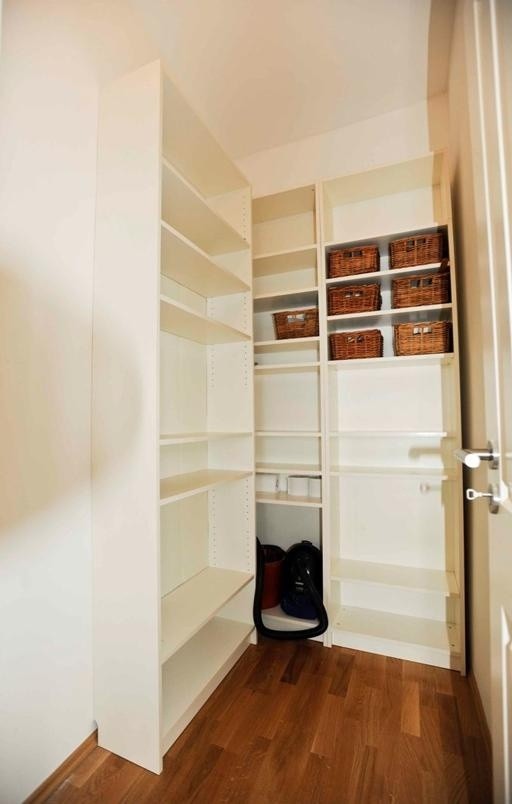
327, 283, 381, 315
328, 329, 383, 361
390, 272, 450, 309
272, 307, 319, 341
392, 320, 453, 357
389, 232, 444, 270
328, 245, 379, 278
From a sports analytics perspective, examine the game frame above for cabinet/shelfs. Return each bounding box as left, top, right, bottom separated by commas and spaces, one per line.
253, 176, 324, 652
318, 145, 469, 673
90, 56, 256, 779
